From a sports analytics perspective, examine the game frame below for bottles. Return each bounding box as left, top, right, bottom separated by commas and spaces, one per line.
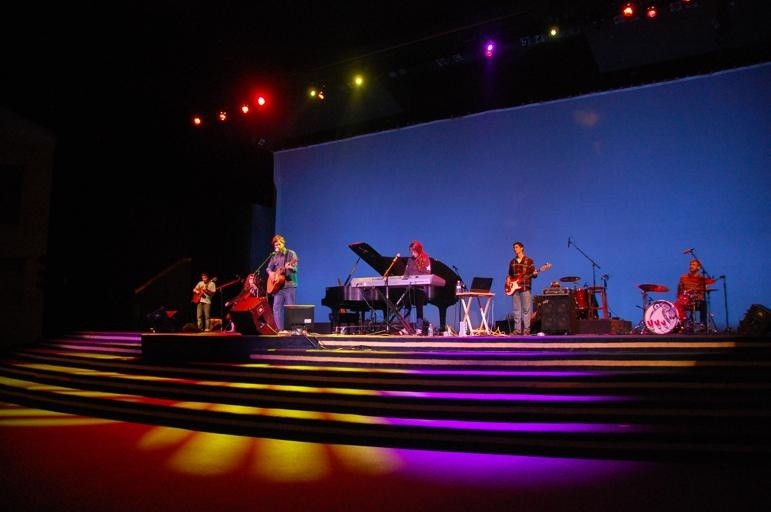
455, 280, 465, 294
427, 323, 433, 337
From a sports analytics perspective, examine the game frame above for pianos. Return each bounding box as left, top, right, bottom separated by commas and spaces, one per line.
322, 241, 467, 332
350, 274, 445, 287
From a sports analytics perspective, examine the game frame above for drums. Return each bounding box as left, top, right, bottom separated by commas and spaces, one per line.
644, 300, 687, 335
563, 287, 589, 311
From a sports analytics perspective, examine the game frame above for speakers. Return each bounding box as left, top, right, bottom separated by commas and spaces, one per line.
230, 300, 279, 335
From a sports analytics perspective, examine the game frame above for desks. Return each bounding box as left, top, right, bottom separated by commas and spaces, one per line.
456, 291, 496, 335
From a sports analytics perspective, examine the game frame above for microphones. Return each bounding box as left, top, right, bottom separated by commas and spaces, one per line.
681, 247, 694, 254
272, 248, 279, 258
567, 236, 570, 248
392, 253, 400, 262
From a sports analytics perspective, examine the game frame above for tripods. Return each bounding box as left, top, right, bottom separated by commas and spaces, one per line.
369, 277, 395, 335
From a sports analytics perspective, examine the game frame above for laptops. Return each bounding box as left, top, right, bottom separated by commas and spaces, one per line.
470, 277, 493, 292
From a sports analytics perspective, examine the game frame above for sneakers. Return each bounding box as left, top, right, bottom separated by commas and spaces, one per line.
415, 327, 424, 337
510, 330, 531, 337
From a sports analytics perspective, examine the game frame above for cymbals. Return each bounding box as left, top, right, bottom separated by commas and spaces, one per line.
695, 288, 720, 293
560, 277, 580, 282
640, 284, 669, 292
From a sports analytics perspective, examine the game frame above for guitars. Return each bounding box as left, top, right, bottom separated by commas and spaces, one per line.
193, 278, 217, 303
505, 263, 551, 296
266, 258, 298, 295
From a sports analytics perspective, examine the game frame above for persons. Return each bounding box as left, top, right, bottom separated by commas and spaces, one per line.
506, 242, 538, 337
265, 234, 298, 332
399, 240, 431, 337
675, 260, 707, 334
192, 273, 216, 332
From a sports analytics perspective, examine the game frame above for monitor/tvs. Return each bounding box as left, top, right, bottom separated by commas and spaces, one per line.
284, 305, 315, 336
